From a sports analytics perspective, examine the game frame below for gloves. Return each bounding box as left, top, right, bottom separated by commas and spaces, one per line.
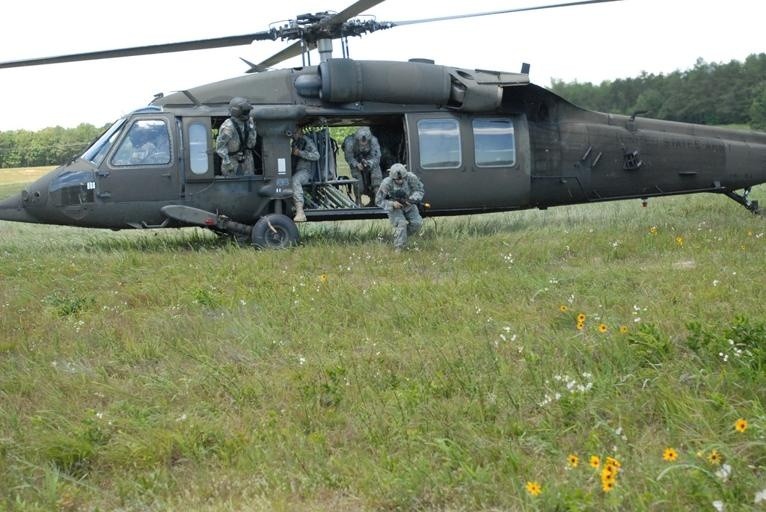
223, 163, 235, 174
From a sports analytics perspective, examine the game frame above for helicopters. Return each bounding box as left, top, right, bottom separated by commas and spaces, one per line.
0, 0, 766, 250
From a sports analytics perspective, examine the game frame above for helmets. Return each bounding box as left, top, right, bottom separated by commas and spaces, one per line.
355, 127, 373, 141
227, 97, 254, 118
387, 163, 408, 179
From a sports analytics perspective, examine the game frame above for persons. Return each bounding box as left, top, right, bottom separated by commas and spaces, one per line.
291, 127, 321, 222
215, 95, 257, 175
113, 127, 158, 165
342, 127, 382, 206
374, 162, 425, 247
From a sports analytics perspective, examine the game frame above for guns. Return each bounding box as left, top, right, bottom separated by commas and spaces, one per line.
385, 193, 430, 213
357, 152, 370, 172
289, 137, 305, 173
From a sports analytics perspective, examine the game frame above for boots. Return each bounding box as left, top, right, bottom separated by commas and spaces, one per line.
356, 196, 365, 208
292, 202, 308, 222
